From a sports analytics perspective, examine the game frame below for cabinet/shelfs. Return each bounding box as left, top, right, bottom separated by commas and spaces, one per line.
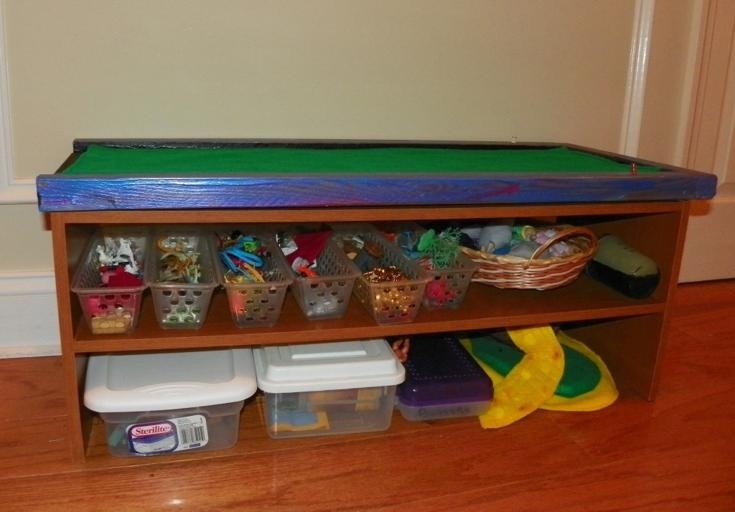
51, 200, 713, 465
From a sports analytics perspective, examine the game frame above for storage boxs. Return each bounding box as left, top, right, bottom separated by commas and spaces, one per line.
252, 339, 407, 440
385, 333, 495, 421
83, 348, 258, 457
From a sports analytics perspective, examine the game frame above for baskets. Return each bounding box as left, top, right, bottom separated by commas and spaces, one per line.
140, 228, 220, 333
207, 230, 294, 332
462, 227, 602, 294
269, 232, 363, 322
67, 224, 148, 335
